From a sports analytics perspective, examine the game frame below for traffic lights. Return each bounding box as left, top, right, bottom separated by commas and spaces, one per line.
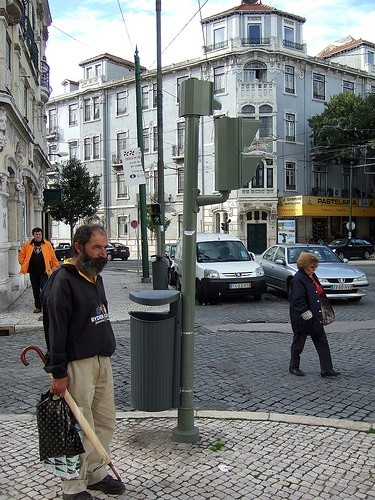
213, 115, 263, 192
221, 223, 224, 230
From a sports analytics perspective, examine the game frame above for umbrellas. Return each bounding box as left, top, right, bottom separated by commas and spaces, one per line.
21, 346, 122, 481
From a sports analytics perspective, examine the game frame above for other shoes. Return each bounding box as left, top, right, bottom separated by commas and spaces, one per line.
289, 367, 305, 376
62, 490, 94, 500
321, 369, 341, 377
33, 308, 41, 313
86, 474, 126, 495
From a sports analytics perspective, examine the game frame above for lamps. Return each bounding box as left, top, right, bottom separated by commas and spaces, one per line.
48, 152, 69, 157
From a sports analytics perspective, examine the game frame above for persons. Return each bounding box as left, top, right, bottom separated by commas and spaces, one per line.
40, 224, 125, 500
18, 228, 59, 313
290, 252, 341, 377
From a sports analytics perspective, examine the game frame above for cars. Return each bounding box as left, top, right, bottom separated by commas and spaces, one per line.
54, 242, 71, 259
165, 243, 177, 285
327, 238, 374, 259
254, 243, 369, 305
105, 243, 130, 261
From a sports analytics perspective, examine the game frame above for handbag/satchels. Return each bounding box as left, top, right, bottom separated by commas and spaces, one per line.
36, 388, 86, 480
320, 298, 335, 326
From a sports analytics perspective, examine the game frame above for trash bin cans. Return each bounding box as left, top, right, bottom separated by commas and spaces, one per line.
151, 255, 169, 290
127, 290, 182, 412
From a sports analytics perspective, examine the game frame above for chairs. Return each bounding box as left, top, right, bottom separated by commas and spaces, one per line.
218, 247, 234, 259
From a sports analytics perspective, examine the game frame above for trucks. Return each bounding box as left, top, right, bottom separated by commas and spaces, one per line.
173, 231, 267, 303
